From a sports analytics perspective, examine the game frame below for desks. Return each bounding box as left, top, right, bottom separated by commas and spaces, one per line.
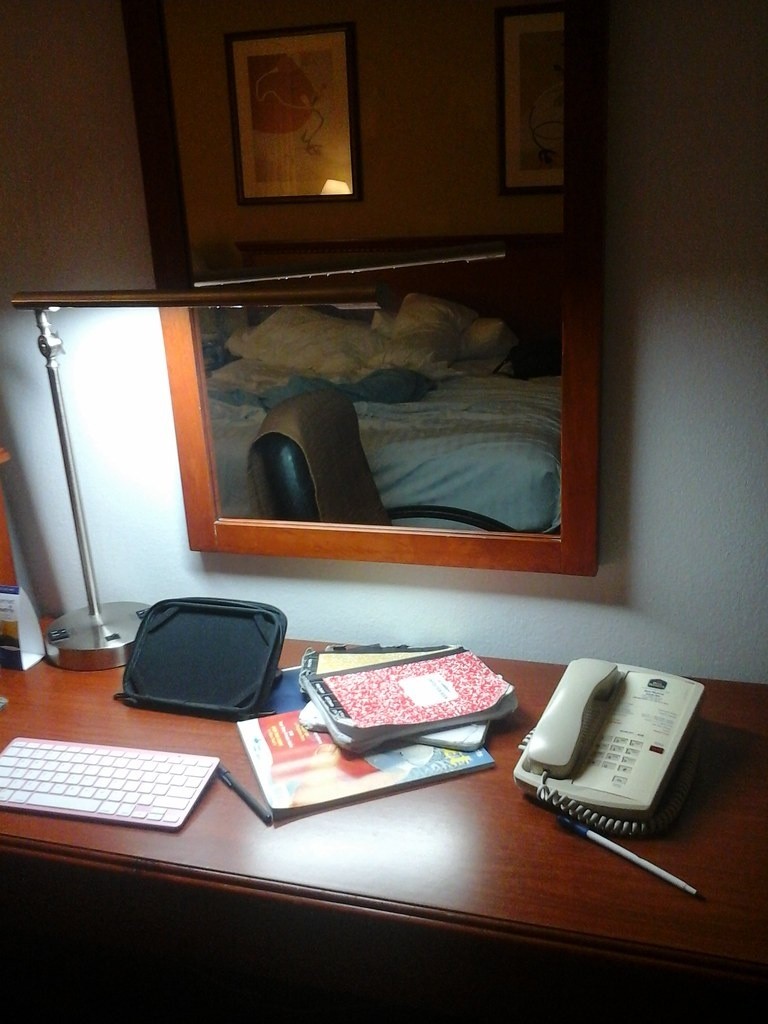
0, 614, 767, 996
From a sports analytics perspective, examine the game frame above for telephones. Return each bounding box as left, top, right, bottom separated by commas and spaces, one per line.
512, 660, 707, 837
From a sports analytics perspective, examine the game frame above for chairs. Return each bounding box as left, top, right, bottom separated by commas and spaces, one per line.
245, 387, 519, 534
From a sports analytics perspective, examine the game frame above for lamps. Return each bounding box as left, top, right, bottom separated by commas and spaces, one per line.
4, 282, 388, 675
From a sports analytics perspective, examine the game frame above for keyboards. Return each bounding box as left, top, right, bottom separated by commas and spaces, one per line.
0, 736, 221, 834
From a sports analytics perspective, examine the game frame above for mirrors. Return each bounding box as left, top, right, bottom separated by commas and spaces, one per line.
120, 0, 606, 582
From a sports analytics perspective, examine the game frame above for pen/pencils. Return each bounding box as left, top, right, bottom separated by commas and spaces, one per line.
555, 814, 699, 896
215, 762, 272, 825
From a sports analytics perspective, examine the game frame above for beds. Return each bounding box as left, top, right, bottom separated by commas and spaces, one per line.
188, 234, 564, 538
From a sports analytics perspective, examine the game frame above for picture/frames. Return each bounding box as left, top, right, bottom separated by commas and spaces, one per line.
492, 2, 566, 195
224, 24, 365, 208
